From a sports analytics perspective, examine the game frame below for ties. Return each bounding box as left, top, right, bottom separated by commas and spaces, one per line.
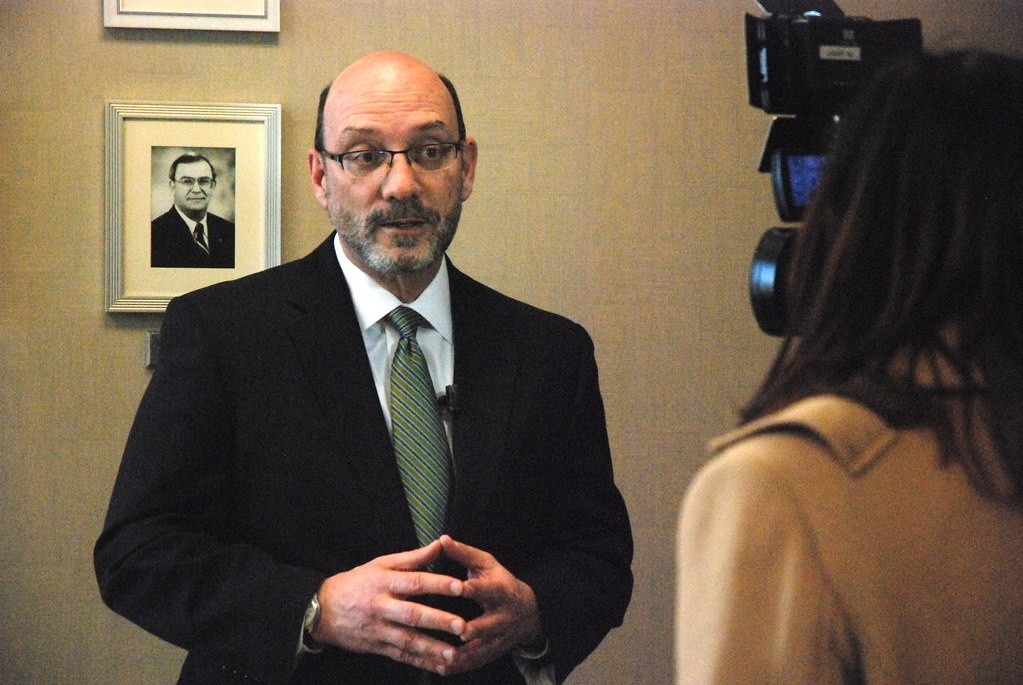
192, 222, 207, 249
381, 305, 455, 573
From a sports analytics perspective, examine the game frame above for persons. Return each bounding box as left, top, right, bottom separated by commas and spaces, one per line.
151, 152, 234, 268
87, 48, 635, 685
675, 45, 1023, 685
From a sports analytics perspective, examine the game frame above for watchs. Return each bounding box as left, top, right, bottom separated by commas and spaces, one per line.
303, 594, 323, 650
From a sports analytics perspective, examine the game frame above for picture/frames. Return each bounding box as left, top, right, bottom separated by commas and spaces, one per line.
102, 0, 281, 33
103, 99, 281, 315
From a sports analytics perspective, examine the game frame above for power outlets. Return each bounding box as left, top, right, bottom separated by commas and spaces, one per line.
144, 330, 162, 369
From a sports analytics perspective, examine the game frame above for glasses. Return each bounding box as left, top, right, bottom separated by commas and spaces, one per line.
174, 176, 213, 187
320, 141, 464, 180
